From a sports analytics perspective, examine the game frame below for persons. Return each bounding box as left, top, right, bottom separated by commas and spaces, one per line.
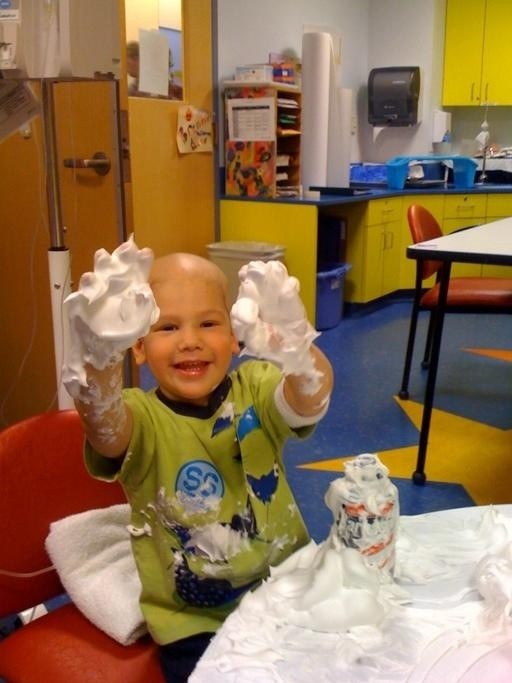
73, 241, 334, 682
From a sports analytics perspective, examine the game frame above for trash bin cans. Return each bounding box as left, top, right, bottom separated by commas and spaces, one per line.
204, 238, 286, 312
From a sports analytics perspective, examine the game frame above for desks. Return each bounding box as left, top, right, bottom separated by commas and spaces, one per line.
408, 214, 511, 485
186, 503, 512, 683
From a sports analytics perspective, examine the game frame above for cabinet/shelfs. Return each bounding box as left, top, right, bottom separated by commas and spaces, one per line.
441, 0, 512, 109
223, 80, 302, 199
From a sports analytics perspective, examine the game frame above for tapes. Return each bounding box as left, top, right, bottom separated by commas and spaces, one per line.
303, 190, 321, 198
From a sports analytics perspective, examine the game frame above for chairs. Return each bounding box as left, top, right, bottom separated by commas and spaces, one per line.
396, 204, 511, 400
0, 408, 167, 682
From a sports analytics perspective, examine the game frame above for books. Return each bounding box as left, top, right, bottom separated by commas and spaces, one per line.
276, 154, 291, 169
277, 172, 289, 183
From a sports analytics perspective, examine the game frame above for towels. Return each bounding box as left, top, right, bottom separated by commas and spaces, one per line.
44, 502, 148, 646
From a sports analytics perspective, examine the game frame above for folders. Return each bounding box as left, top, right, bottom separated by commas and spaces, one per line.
309, 185, 372, 197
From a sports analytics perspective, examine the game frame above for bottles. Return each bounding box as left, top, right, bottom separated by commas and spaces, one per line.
333, 452, 400, 585
442, 129, 452, 155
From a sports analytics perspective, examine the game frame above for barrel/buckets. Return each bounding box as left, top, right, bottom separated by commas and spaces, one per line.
316, 261, 353, 330
